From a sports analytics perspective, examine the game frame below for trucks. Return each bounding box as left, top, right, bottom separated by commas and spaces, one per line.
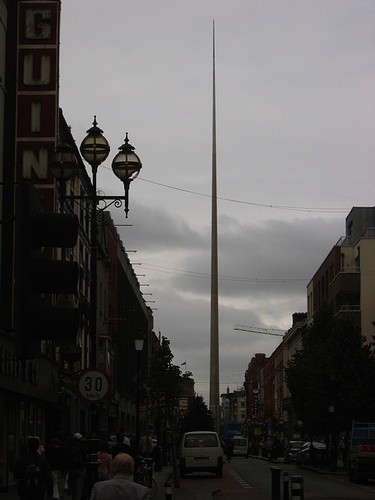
347, 422, 375, 485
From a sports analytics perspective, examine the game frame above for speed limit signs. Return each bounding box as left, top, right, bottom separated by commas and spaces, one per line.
77, 370, 110, 403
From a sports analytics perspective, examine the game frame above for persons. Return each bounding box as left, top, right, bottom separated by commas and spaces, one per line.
224, 438, 234, 463
13, 433, 54, 500
258, 432, 290, 465
63, 425, 180, 500
297, 435, 350, 470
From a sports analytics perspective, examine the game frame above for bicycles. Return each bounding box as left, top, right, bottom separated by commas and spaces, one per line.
135, 457, 158, 493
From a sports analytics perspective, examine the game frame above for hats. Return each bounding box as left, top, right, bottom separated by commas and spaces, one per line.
73, 432, 83, 440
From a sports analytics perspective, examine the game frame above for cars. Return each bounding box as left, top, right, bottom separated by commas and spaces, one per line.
283, 441, 326, 465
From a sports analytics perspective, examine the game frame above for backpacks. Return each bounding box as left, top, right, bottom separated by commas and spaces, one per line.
23, 454, 45, 493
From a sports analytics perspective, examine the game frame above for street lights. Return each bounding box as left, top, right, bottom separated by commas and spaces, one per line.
48, 115, 145, 499
132, 328, 145, 476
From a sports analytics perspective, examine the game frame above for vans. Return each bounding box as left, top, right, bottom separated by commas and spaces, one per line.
229, 435, 249, 459
177, 431, 223, 479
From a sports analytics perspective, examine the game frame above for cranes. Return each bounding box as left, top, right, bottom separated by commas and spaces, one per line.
233, 323, 288, 337
